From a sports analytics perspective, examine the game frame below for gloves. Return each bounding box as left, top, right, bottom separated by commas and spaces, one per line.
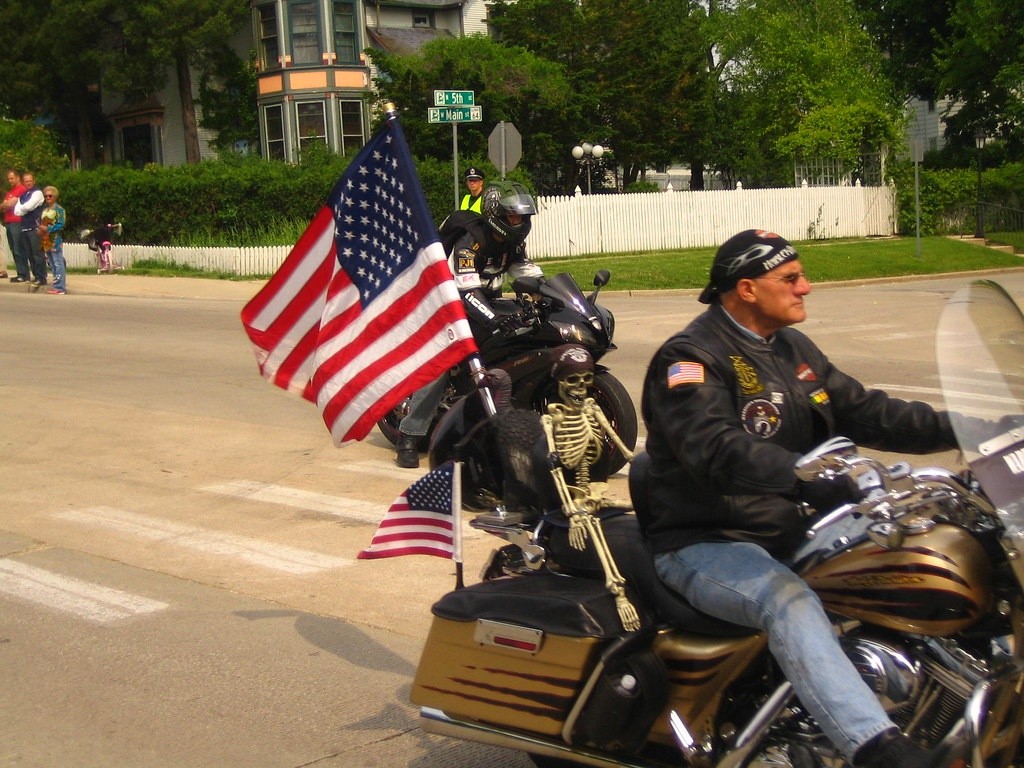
489, 314, 521, 336
798, 476, 866, 519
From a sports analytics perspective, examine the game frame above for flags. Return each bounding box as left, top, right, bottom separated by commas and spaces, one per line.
241, 118, 479, 447
357, 460, 465, 561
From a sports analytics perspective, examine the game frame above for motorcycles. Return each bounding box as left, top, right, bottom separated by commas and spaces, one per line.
409, 277, 1024, 768
377, 269, 638, 481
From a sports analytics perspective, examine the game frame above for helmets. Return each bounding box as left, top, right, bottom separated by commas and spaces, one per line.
480, 181, 536, 245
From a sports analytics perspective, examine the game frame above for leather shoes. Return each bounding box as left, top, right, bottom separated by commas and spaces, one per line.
397, 431, 420, 468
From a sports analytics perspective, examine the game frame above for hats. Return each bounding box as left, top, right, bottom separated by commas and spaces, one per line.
464, 167, 485, 181
698, 229, 798, 305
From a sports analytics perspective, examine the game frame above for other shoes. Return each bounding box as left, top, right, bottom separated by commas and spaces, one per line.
48, 290, 65, 294
866, 735, 966, 768
11, 277, 30, 282
33, 279, 47, 285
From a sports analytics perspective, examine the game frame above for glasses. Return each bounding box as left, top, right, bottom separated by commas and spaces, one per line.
44, 195, 54, 198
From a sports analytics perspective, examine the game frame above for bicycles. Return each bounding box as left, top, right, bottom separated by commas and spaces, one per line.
81, 222, 126, 273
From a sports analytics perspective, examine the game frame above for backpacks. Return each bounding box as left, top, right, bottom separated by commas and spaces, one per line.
437, 210, 487, 274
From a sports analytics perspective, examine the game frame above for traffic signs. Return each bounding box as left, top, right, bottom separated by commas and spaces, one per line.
434, 90, 476, 107
428, 105, 482, 123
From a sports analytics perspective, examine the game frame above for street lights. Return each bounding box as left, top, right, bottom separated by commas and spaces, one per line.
572, 142, 604, 195
972, 128, 988, 239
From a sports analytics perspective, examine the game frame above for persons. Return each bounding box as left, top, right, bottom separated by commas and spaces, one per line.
0, 168, 30, 282
35, 186, 66, 295
0, 189, 9, 278
13, 171, 49, 287
460, 167, 486, 214
396, 180, 544, 469
636, 229, 1024, 768
